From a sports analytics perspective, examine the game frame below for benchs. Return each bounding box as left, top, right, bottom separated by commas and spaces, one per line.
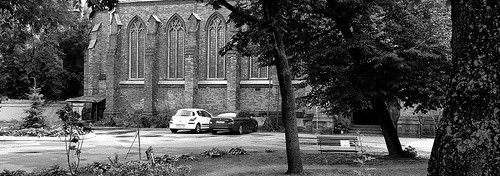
317, 136, 363, 161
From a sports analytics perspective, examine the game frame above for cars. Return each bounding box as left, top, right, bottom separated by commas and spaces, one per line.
169, 108, 214, 133
209, 110, 258, 134
